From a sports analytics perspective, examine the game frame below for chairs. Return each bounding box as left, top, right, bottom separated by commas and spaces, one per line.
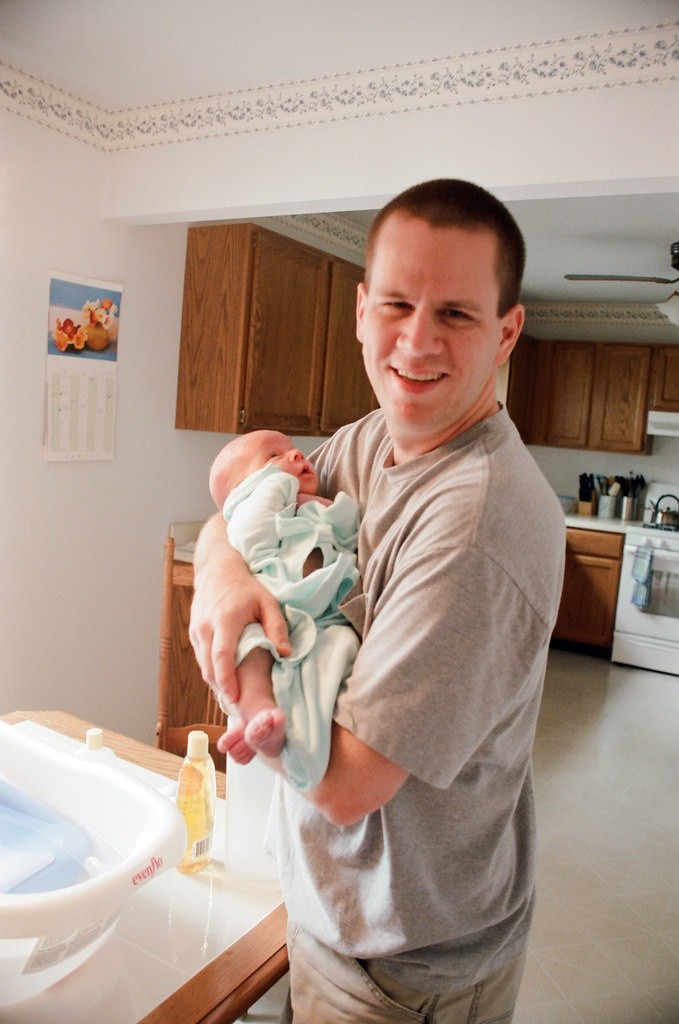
155, 538, 232, 755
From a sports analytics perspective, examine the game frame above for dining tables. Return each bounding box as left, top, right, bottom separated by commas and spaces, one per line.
0, 712, 293, 1024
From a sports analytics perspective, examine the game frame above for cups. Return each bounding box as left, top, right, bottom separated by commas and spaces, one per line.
619, 494, 638, 521
598, 492, 617, 518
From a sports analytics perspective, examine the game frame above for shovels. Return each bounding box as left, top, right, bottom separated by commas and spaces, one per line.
596, 473, 621, 497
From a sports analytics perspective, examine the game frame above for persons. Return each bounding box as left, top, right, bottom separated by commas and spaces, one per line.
206, 429, 361, 770
183, 176, 575, 1023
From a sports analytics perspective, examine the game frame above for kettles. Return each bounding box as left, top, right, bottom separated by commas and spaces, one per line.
649, 493, 679, 528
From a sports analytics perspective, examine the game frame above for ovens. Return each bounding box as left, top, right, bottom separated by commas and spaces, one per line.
610, 526, 678, 678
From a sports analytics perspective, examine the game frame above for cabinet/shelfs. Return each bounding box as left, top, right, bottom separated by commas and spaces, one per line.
549, 527, 624, 656
177, 220, 383, 440
498, 334, 678, 458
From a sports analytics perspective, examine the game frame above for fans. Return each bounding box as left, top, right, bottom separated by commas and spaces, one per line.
560, 241, 679, 330
